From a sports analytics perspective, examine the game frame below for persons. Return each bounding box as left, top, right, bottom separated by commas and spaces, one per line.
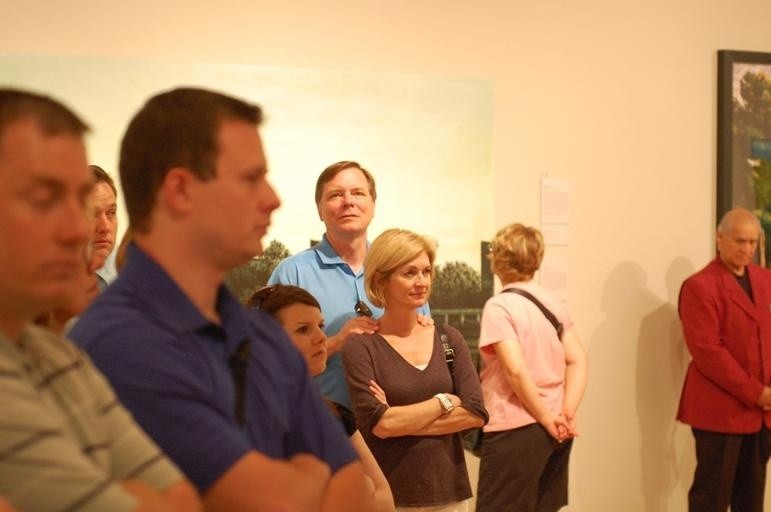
0, 83, 205, 512
337, 225, 489, 511
62, 86, 382, 511
38, 200, 100, 336
79, 160, 125, 325
246, 279, 400, 512
472, 222, 591, 512
670, 204, 771, 512
264, 158, 432, 412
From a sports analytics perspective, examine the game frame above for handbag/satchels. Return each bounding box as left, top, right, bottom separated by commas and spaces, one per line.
460, 426, 483, 459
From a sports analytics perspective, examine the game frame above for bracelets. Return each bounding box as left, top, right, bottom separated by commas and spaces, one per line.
436, 391, 456, 416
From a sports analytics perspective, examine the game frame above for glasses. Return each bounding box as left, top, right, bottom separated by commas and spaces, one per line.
355, 300, 373, 317
250, 283, 280, 313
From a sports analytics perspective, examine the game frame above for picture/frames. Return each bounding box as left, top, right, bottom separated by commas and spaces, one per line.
717, 49, 771, 271
